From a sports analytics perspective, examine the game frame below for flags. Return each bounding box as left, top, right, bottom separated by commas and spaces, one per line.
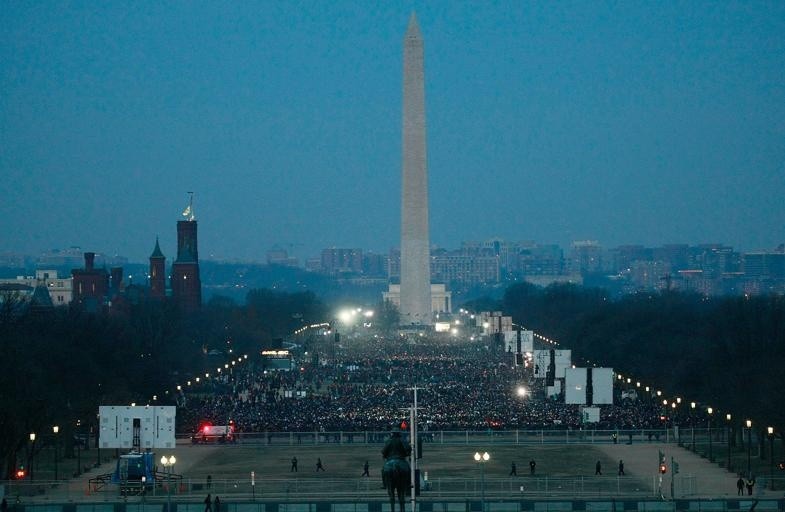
182, 205, 190, 216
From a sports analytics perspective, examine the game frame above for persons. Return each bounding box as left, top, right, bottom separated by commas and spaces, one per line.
617, 459, 626, 476
289, 456, 298, 473
737, 475, 746, 495
315, 457, 326, 473
360, 460, 371, 477
379, 430, 413, 490
202, 492, 213, 511
206, 473, 212, 490
527, 456, 538, 476
176, 308, 718, 444
509, 460, 518, 478
213, 495, 221, 512
594, 458, 603, 475
746, 475, 758, 496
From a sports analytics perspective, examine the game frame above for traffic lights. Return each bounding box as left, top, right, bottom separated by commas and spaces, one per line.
400, 418, 408, 430
672, 461, 680, 475
661, 454, 666, 463
16, 469, 25, 478
660, 465, 667, 473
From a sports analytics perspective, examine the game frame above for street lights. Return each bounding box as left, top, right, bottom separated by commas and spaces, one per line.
96, 412, 101, 465
129, 353, 248, 418
459, 307, 683, 447
706, 406, 714, 463
51, 424, 60, 486
746, 418, 752, 480
767, 424, 776, 490
690, 402, 697, 453
726, 412, 732, 472
295, 321, 331, 344
28, 431, 35, 494
160, 454, 177, 511
474, 450, 489, 511
74, 417, 81, 474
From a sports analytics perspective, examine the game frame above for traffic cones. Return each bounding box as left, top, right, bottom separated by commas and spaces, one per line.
91, 482, 99, 492
85, 488, 91, 496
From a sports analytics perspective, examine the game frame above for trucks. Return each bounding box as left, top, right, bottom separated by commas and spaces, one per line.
191, 424, 234, 442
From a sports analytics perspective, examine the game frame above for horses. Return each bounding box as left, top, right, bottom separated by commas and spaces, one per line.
383, 458, 411, 512
